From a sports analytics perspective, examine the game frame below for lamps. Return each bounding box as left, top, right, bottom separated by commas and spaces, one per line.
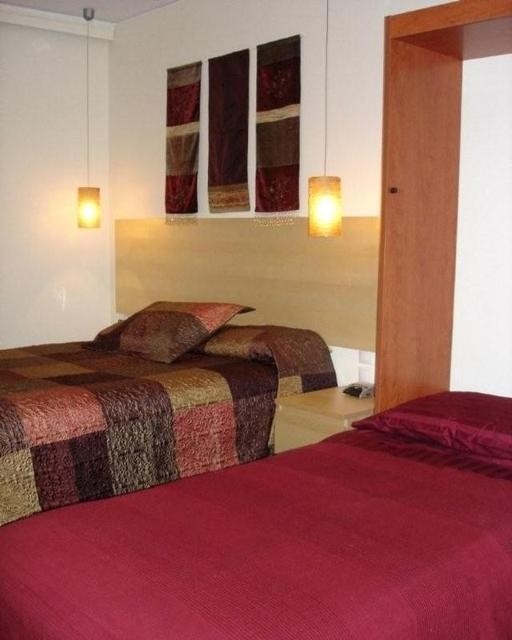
306, 1, 344, 239
76, 7, 102, 230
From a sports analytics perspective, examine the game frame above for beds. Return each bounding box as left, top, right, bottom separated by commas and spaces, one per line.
1, 390, 512, 640
0, 337, 338, 525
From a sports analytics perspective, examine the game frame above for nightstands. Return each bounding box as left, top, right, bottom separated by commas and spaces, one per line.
273, 385, 374, 454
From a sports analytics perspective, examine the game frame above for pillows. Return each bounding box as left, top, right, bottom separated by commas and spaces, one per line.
81, 301, 258, 365
351, 390, 512, 464
